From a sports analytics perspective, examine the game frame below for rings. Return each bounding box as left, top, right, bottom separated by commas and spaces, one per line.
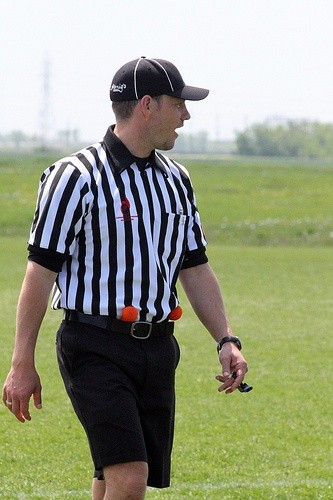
6, 400, 13, 405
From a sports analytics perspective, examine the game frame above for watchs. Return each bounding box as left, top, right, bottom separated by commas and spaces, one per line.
216, 336, 242, 356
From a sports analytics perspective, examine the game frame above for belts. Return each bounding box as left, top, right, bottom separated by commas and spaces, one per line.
61, 308, 177, 337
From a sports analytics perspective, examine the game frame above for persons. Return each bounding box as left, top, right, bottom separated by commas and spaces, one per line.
1, 54, 248, 499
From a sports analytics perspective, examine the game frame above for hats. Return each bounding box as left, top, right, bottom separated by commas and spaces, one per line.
110, 56, 210, 100
